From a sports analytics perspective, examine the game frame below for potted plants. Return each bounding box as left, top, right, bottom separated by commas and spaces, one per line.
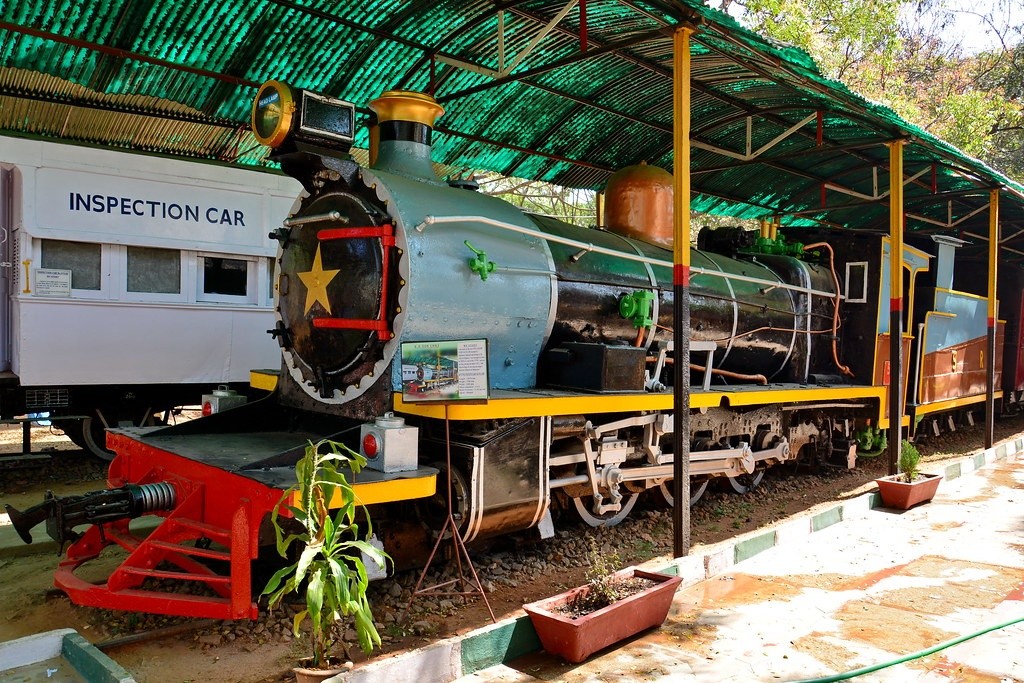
873, 439, 944, 510
258, 436, 396, 683
522, 534, 684, 662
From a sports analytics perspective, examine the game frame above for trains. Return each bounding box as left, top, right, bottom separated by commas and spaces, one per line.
2, 63, 1024, 620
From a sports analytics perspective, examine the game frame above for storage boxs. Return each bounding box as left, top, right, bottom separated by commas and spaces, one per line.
555, 340, 647, 394
